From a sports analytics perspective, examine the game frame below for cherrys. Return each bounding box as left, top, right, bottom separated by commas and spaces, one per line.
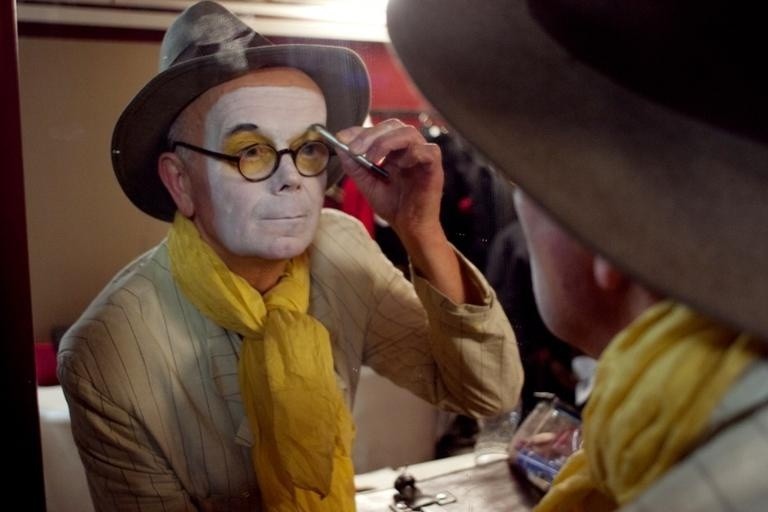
315, 126, 391, 180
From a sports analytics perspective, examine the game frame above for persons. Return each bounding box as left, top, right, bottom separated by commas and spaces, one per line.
387, 0, 768, 510
56, 3, 528, 512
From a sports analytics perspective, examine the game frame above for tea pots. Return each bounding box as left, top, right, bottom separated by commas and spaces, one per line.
0, 0, 601, 512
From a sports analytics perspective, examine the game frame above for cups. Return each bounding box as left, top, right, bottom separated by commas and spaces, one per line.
170, 139, 331, 181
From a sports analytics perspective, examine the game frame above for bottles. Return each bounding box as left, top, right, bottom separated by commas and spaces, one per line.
385, 0, 767, 344
110, 0, 372, 222
